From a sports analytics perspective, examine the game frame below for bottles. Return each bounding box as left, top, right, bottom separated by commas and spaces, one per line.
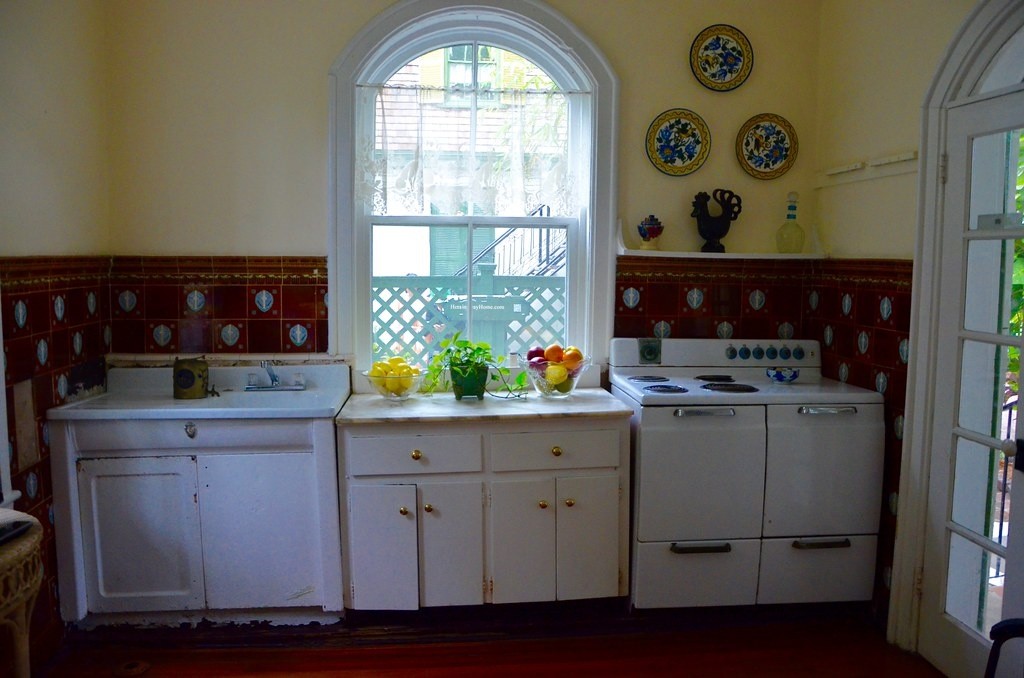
777, 192, 804, 253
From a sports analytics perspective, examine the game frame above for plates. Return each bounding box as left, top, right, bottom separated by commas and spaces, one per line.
736, 113, 798, 180
646, 109, 711, 176
689, 24, 754, 91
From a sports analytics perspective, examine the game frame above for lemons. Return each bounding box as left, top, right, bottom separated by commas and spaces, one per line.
546, 366, 568, 384
368, 356, 419, 395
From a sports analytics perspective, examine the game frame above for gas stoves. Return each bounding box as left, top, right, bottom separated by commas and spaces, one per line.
609, 338, 884, 406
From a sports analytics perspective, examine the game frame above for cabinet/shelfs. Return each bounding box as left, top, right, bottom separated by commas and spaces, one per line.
349, 433, 484, 609
490, 428, 622, 604
75, 451, 321, 612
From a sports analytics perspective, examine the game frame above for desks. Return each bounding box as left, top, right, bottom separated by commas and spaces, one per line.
0, 519, 44, 678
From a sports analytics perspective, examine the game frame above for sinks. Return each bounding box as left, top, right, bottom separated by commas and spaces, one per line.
199, 388, 321, 419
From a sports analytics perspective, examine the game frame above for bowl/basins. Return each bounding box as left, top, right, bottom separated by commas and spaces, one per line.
766, 369, 799, 384
362, 369, 428, 402
517, 356, 592, 398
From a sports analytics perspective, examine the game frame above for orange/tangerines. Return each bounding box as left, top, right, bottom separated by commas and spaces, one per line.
544, 345, 563, 362
562, 351, 581, 369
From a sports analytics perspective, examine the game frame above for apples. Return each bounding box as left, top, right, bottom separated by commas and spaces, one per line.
563, 346, 583, 359
555, 377, 573, 392
534, 378, 553, 393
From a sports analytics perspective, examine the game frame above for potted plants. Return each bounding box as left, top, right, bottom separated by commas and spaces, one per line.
419, 330, 529, 401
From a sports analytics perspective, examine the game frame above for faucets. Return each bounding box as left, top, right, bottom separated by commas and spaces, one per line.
261, 359, 281, 388
